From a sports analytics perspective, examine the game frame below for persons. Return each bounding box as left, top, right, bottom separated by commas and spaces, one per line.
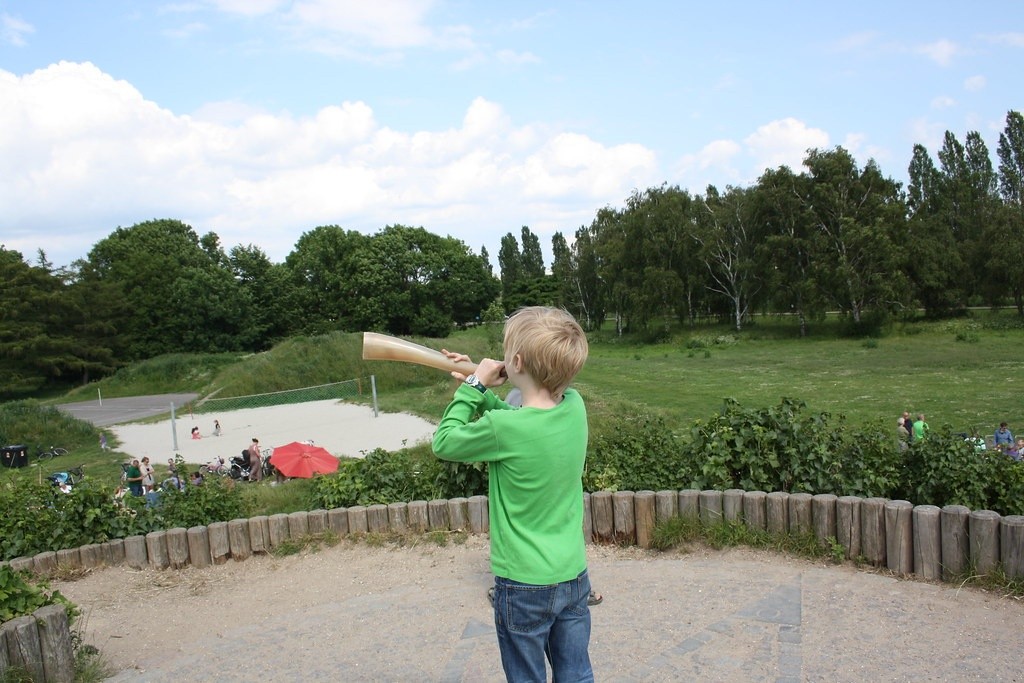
897, 412, 929, 454
115, 457, 205, 510
212, 419, 221, 437
59, 482, 71, 493
191, 426, 202, 439
431, 306, 603, 683
248, 439, 264, 482
99, 432, 110, 452
964, 422, 1024, 461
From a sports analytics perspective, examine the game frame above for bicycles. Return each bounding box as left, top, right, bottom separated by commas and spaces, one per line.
261, 448, 275, 478
198, 456, 229, 477
37, 446, 69, 462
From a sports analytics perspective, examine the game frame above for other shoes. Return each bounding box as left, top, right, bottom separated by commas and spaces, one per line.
587, 591, 603, 605
487, 586, 495, 607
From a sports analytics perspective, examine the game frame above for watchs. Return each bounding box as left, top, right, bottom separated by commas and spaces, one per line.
465, 374, 487, 394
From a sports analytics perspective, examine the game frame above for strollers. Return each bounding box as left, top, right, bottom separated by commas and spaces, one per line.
228, 449, 254, 481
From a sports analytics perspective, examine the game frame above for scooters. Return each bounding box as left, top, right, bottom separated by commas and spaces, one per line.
45, 463, 85, 502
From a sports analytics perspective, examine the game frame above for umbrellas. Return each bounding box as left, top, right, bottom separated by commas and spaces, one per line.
269, 442, 339, 477
